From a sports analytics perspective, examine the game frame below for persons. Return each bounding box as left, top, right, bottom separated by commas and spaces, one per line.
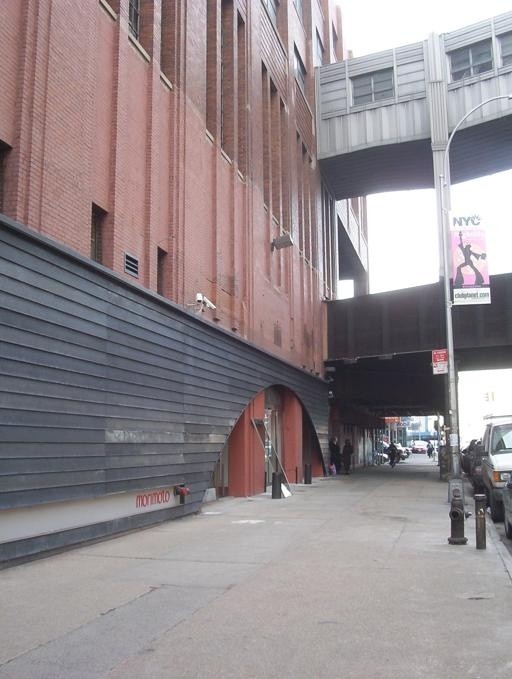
386, 442, 398, 467
342, 438, 354, 474
452, 231, 487, 288
328, 435, 342, 474
426, 441, 434, 459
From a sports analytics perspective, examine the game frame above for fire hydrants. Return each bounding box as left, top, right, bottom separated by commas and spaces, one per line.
447, 493, 469, 545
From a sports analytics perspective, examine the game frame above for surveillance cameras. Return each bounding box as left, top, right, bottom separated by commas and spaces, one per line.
187, 292, 202, 307
328, 390, 333, 397
198, 296, 216, 314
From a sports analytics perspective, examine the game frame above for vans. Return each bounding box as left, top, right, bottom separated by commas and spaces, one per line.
481, 414, 512, 522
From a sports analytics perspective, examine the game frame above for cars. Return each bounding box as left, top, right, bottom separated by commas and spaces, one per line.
380, 441, 410, 461
413, 439, 428, 454
502, 474, 512, 539
461, 438, 483, 489
